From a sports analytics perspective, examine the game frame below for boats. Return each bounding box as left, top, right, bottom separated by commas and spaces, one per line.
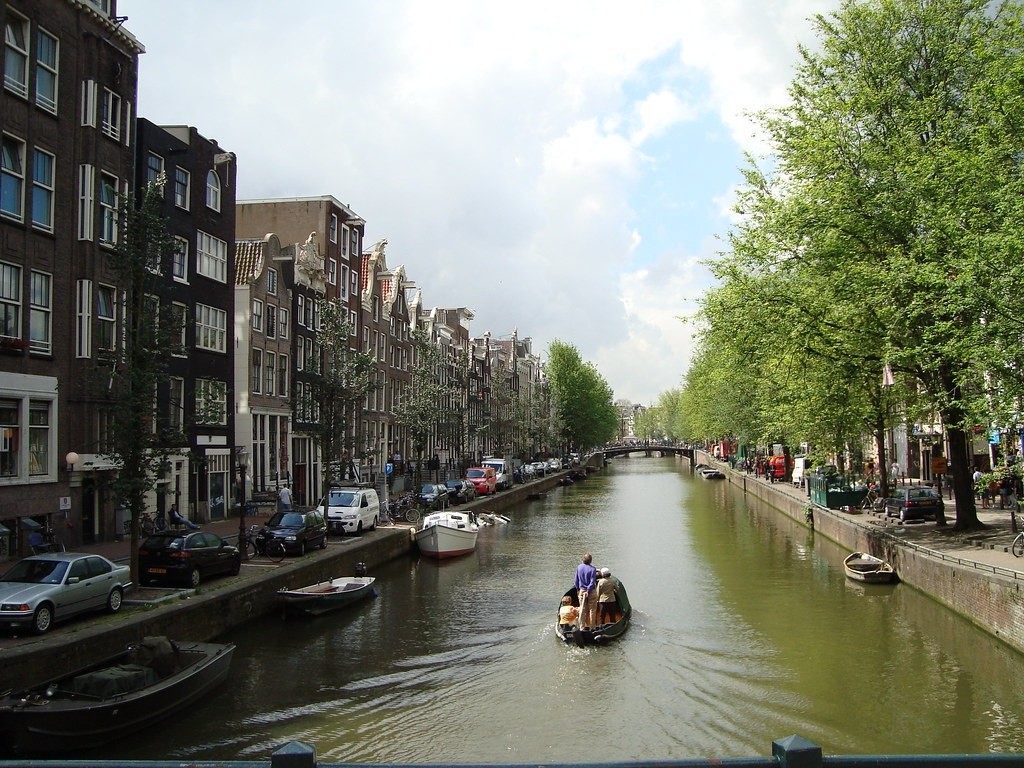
695, 463, 711, 474
555, 568, 632, 646
843, 551, 895, 583
410, 510, 479, 560
476, 512, 512, 526
701, 470, 725, 479
0, 633, 237, 759
275, 562, 377, 619
558, 465, 601, 486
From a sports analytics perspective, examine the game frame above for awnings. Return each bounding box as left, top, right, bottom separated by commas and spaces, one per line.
21, 519, 41, 530
0, 523, 11, 535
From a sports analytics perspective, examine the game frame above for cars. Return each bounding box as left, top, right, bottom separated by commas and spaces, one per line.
254, 508, 328, 558
0, 552, 132, 635
444, 479, 476, 505
416, 483, 449, 510
883, 486, 945, 522
520, 453, 580, 478
769, 455, 795, 480
138, 529, 241, 588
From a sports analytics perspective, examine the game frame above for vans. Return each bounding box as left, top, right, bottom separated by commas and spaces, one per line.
315, 486, 380, 537
709, 444, 720, 459
460, 467, 497, 496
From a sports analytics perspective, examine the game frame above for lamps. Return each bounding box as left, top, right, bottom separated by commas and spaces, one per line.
66, 451, 79, 473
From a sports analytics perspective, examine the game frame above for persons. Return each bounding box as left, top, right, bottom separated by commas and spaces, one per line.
279, 483, 293, 509
891, 458, 900, 483
752, 457, 760, 478
559, 596, 579, 632
981, 468, 1024, 509
746, 458, 752, 475
596, 567, 620, 625
865, 458, 878, 478
574, 552, 598, 631
972, 467, 982, 500
170, 504, 201, 530
393, 450, 402, 460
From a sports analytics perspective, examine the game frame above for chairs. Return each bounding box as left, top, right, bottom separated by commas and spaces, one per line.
168, 511, 182, 529
28, 532, 51, 556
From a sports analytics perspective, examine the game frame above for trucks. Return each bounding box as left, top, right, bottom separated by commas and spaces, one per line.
480, 459, 508, 490
718, 441, 738, 463
791, 458, 813, 488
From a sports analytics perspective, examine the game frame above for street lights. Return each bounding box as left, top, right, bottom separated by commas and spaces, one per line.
237, 448, 250, 563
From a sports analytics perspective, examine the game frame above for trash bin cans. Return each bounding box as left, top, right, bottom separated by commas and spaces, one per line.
20, 518, 41, 558
0, 523, 11, 564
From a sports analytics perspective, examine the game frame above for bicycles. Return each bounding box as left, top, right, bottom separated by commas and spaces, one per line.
859, 488, 885, 513
379, 490, 421, 526
1011, 513, 1024, 558
514, 462, 532, 485
234, 523, 286, 563
137, 508, 171, 540
48, 527, 66, 553
